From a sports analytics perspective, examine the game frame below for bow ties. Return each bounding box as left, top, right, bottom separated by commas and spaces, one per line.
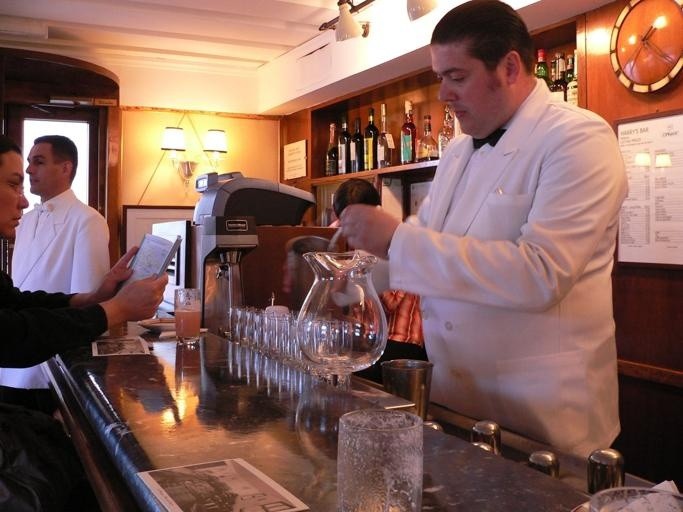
472, 129, 508, 148
34, 201, 54, 212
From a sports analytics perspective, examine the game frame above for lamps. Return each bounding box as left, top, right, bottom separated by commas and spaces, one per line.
407, 0, 438, 22
160, 126, 228, 182
336, 0, 364, 42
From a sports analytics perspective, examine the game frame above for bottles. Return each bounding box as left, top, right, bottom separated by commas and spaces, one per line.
323, 99, 454, 177
533, 48, 578, 106
467, 420, 623, 494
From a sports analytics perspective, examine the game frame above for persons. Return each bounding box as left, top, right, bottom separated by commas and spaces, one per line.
336, 0, 631, 464
0, 134, 112, 512
330, 177, 426, 386
1, 132, 169, 367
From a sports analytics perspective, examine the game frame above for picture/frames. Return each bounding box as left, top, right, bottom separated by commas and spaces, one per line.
613, 108, 683, 270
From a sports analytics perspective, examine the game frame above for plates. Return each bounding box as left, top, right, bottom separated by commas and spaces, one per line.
137, 318, 175, 334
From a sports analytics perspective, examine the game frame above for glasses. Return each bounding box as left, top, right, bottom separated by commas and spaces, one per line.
6, 182, 25, 197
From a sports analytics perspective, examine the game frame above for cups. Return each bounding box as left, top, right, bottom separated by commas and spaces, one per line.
174, 342, 202, 402
588, 486, 683, 512
173, 287, 201, 343
380, 358, 434, 422
228, 341, 324, 413
336, 408, 424, 511
227, 304, 352, 393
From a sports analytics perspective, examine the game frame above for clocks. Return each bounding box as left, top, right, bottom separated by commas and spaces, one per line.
609, 0, 683, 95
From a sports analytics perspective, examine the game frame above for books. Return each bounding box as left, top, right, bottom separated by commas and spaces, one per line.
114, 233, 183, 307
